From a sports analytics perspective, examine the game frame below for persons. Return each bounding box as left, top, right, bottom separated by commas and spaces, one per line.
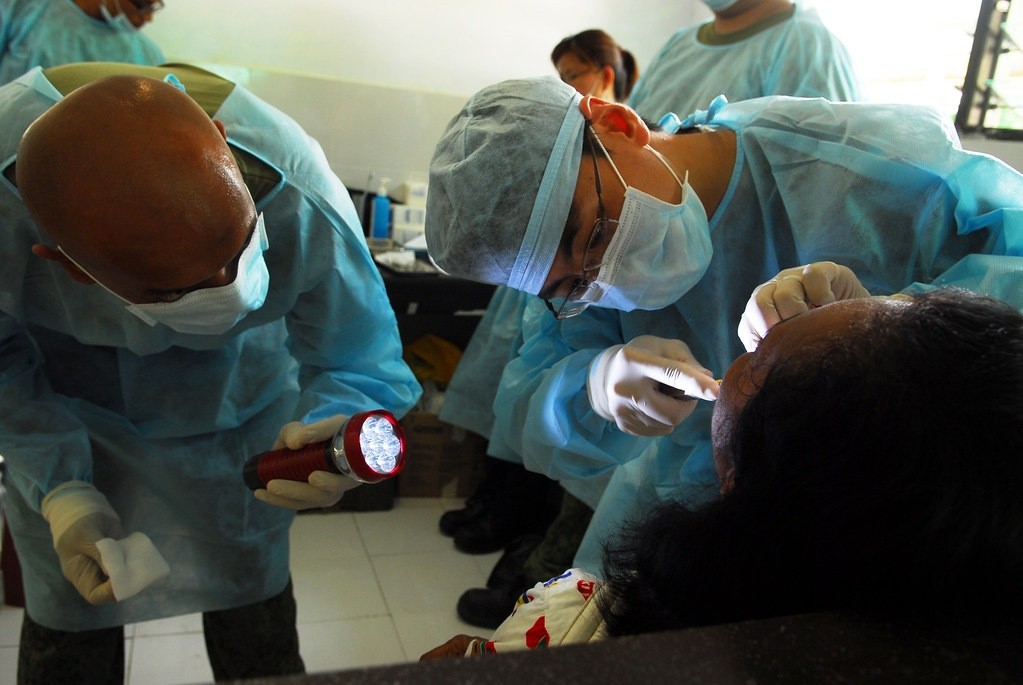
421, 288, 1023, 685
0, 0, 163, 91
0, 63, 420, 685
426, 77, 1023, 575
440, 27, 637, 555
454, 0, 863, 629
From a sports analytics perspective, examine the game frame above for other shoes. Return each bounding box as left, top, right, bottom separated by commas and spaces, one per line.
437, 505, 522, 555
457, 588, 512, 631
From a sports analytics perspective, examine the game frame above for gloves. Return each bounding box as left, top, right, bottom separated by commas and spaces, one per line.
253, 415, 363, 509
738, 261, 918, 353
586, 337, 720, 437
41, 480, 149, 604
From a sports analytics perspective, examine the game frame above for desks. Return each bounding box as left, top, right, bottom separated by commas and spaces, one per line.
365, 238, 500, 493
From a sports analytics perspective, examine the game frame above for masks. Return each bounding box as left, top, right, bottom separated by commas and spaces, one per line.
702, 0, 737, 13
578, 127, 713, 312
56, 168, 271, 335
101, 0, 139, 35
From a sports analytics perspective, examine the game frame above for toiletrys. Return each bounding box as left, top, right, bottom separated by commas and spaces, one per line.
368, 175, 392, 243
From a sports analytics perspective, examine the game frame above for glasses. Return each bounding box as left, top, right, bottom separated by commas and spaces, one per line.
545, 123, 621, 320
130, 0, 165, 14
559, 64, 603, 85
